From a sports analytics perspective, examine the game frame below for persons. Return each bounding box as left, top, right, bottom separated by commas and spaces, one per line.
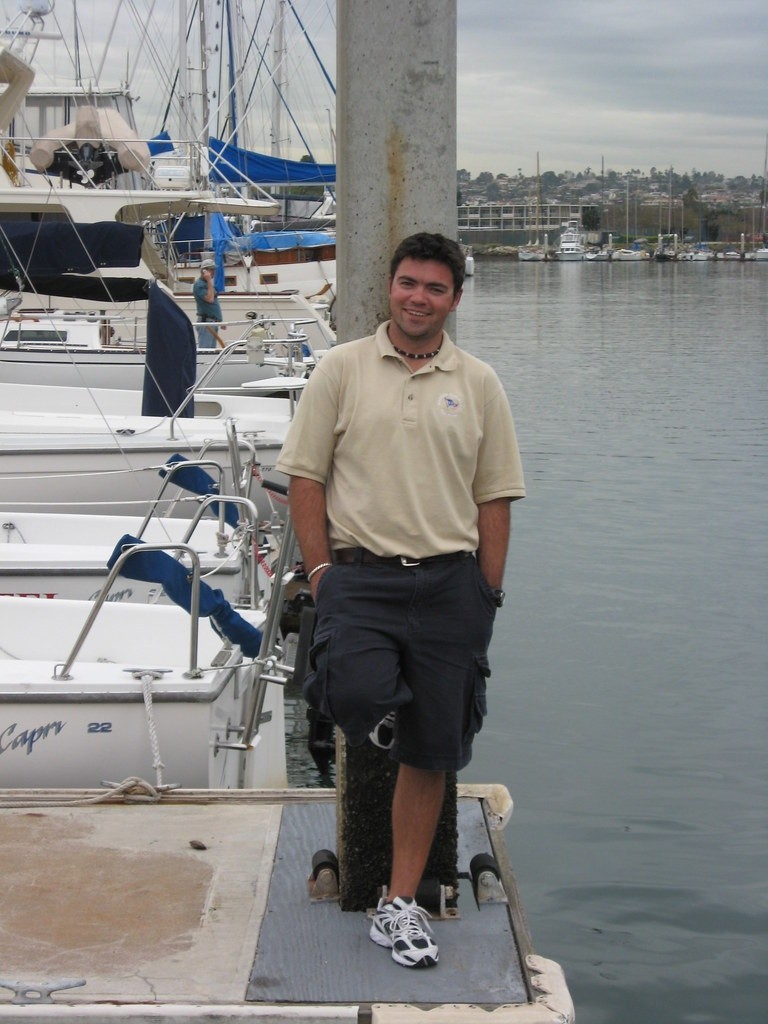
193, 259, 226, 348
275, 231, 528, 968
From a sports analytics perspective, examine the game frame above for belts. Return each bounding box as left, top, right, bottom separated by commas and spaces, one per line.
335, 547, 472, 567
197, 316, 217, 321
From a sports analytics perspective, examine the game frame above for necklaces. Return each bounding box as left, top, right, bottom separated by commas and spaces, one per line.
386, 323, 444, 360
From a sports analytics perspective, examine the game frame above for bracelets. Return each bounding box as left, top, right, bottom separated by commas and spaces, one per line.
491, 585, 505, 608
307, 563, 333, 582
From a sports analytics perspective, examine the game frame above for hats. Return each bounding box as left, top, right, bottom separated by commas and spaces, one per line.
201, 259, 216, 269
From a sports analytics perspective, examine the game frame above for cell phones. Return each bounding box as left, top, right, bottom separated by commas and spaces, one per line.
203, 270, 207, 274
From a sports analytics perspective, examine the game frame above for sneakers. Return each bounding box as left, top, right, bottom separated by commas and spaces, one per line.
369, 710, 397, 750
370, 885, 439, 968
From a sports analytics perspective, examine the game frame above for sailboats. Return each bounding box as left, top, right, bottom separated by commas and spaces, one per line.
519, 146, 768, 262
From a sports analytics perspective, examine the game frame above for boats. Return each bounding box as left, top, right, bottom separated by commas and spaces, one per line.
0, 0, 338, 782
